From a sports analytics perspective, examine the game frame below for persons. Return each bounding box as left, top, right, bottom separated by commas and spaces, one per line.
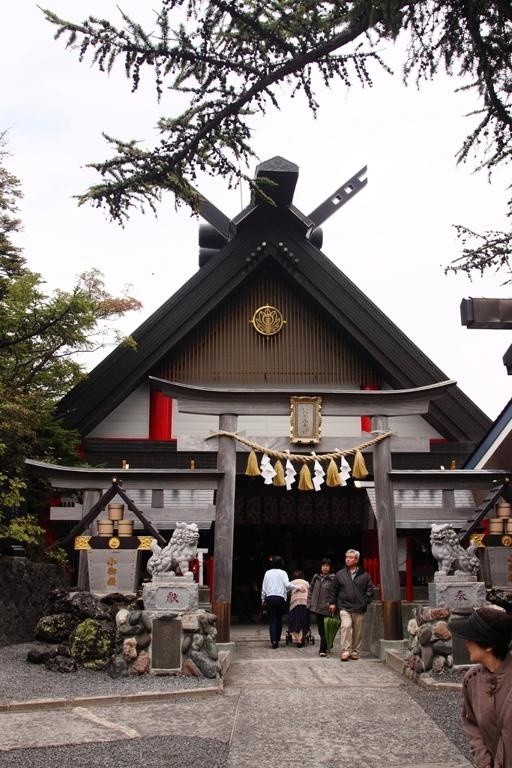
259, 556, 306, 650
287, 570, 310, 649
447, 607, 511, 767
327, 548, 373, 661
306, 559, 337, 657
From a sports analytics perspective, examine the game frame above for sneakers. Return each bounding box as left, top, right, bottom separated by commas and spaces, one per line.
270, 636, 306, 649
318, 651, 327, 657
340, 650, 359, 662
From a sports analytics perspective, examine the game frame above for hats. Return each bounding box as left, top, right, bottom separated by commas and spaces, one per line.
447, 609, 512, 650
